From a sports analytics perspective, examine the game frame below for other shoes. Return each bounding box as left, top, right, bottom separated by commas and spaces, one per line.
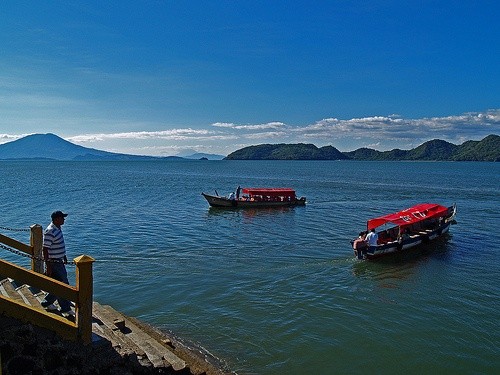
59, 309, 75, 315
43, 304, 57, 310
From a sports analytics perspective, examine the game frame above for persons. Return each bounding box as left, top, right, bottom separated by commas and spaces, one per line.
235, 186, 242, 200
42, 211, 75, 316
242, 194, 298, 202
359, 228, 379, 246
228, 192, 235, 200
382, 219, 440, 241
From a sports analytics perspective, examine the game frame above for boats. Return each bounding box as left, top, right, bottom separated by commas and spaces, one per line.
201, 187, 306, 208
349, 202, 457, 260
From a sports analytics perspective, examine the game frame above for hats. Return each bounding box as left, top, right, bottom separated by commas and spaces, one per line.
51, 211, 68, 221
